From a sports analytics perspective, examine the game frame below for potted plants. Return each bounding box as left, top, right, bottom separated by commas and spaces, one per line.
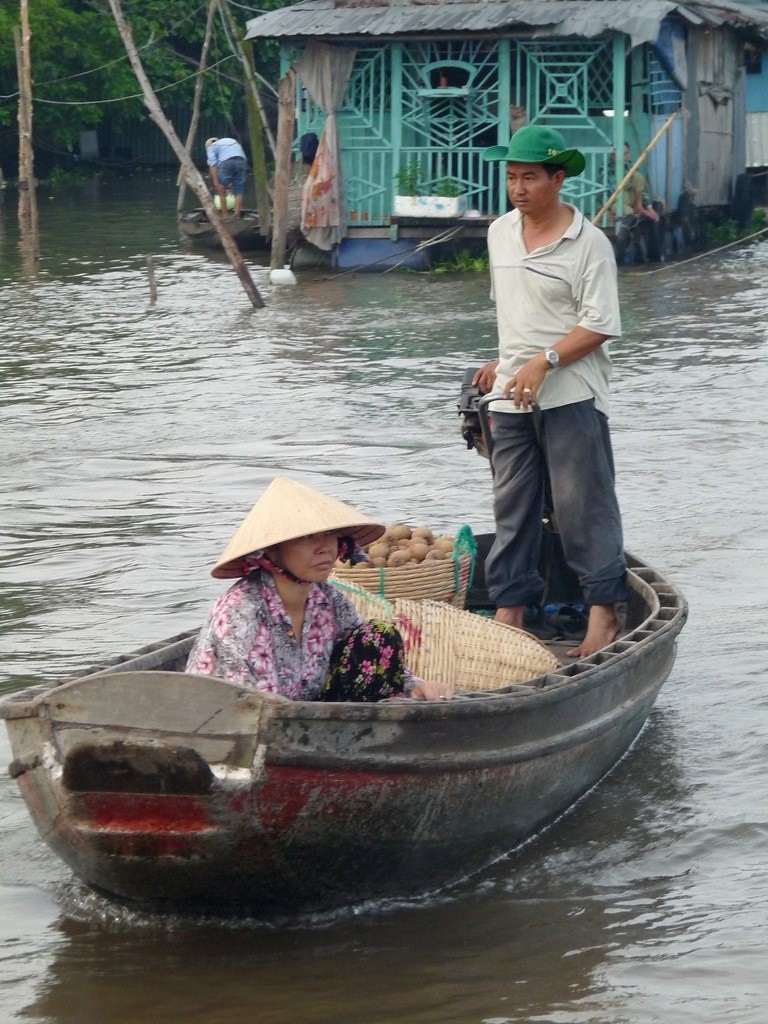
394, 159, 467, 217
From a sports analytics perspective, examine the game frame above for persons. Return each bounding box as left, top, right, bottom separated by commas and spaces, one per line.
471, 125, 631, 660
183, 475, 451, 704
203, 137, 247, 218
596, 141, 661, 223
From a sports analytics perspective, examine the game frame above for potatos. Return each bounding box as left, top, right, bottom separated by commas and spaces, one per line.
335, 523, 455, 569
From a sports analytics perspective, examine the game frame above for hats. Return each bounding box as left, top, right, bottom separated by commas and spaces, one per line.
483, 125, 585, 177
211, 476, 385, 580
206, 138, 217, 147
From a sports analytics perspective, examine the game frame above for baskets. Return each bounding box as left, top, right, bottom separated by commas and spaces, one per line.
328, 577, 561, 696
332, 524, 478, 610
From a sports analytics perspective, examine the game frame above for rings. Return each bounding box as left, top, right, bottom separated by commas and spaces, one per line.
522, 388, 530, 393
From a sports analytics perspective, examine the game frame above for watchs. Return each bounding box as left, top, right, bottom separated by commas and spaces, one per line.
543, 347, 560, 369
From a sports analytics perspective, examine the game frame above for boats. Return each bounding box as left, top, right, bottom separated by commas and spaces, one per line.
0, 367, 688, 919
180, 202, 261, 253
